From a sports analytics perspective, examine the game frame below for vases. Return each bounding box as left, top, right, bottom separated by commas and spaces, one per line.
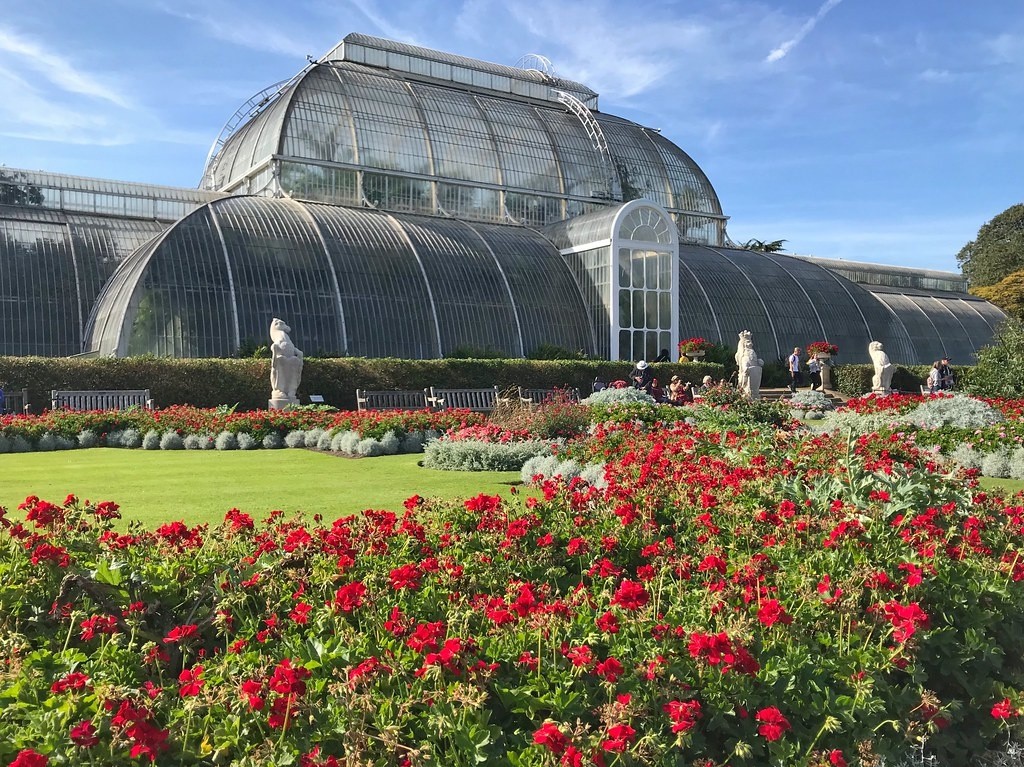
817, 352, 832, 359
685, 350, 705, 356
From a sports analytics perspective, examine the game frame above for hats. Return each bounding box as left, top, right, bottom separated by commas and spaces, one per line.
661, 348, 670, 355
637, 361, 648, 370
942, 356, 951, 360
671, 375, 679, 381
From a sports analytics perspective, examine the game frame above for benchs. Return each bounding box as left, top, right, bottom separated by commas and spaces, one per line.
50, 389, 154, 412
0, 389, 31, 416
518, 386, 582, 407
354, 386, 510, 416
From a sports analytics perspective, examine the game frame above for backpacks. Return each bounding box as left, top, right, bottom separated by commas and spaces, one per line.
785, 355, 796, 366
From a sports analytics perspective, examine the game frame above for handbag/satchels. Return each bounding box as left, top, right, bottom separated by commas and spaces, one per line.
928, 377, 933, 390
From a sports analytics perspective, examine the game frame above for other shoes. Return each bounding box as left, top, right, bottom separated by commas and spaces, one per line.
788, 384, 791, 390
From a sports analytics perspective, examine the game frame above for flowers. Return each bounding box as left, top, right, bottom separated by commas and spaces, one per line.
678, 338, 714, 354
806, 342, 838, 357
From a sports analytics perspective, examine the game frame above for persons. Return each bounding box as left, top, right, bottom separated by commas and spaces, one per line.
930, 356, 954, 393
594, 377, 606, 391
629, 360, 728, 407
789, 347, 802, 394
806, 352, 823, 391
563, 382, 573, 401
